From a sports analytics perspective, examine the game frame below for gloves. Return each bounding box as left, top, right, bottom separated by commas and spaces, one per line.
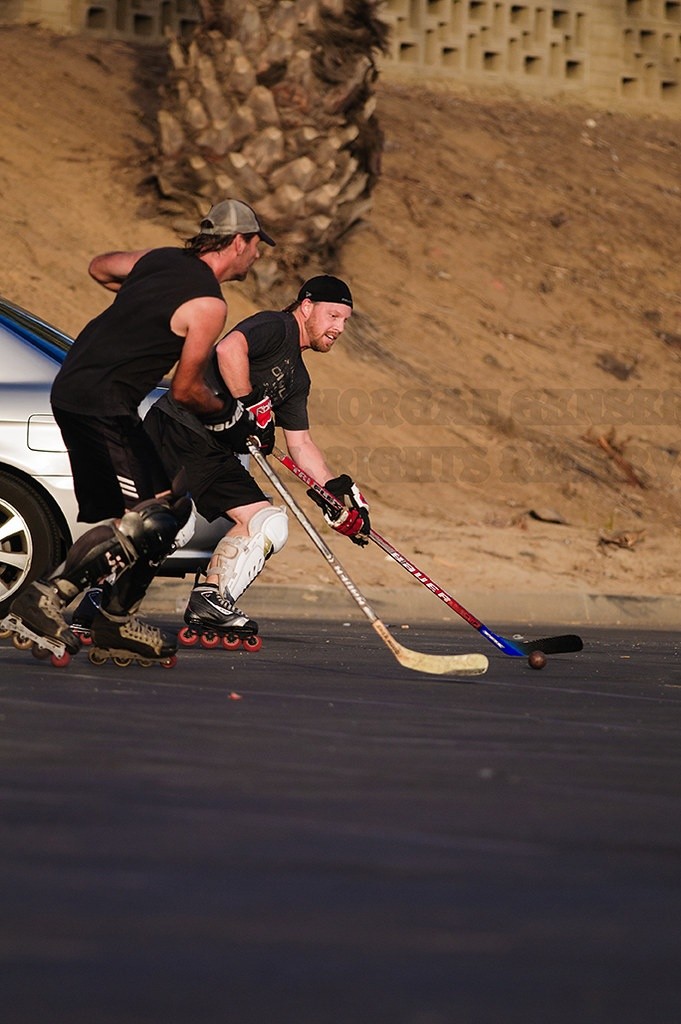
232, 382, 277, 457
198, 392, 263, 455
306, 473, 371, 549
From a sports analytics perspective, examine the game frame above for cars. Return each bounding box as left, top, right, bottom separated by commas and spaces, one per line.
1, 300, 272, 634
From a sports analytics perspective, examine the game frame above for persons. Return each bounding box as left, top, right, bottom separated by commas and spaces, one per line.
1, 197, 275, 665
66, 273, 371, 653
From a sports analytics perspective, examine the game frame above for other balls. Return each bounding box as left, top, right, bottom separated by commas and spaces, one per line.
528, 651, 547, 668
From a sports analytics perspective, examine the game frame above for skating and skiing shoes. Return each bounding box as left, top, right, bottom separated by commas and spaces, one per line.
0, 573, 93, 668
89, 580, 180, 669
177, 566, 263, 652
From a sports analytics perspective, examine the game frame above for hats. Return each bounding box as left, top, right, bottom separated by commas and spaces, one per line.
281, 274, 353, 314
200, 197, 277, 247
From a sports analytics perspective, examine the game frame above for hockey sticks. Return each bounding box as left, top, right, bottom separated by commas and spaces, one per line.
244, 437, 490, 675
272, 445, 584, 657
